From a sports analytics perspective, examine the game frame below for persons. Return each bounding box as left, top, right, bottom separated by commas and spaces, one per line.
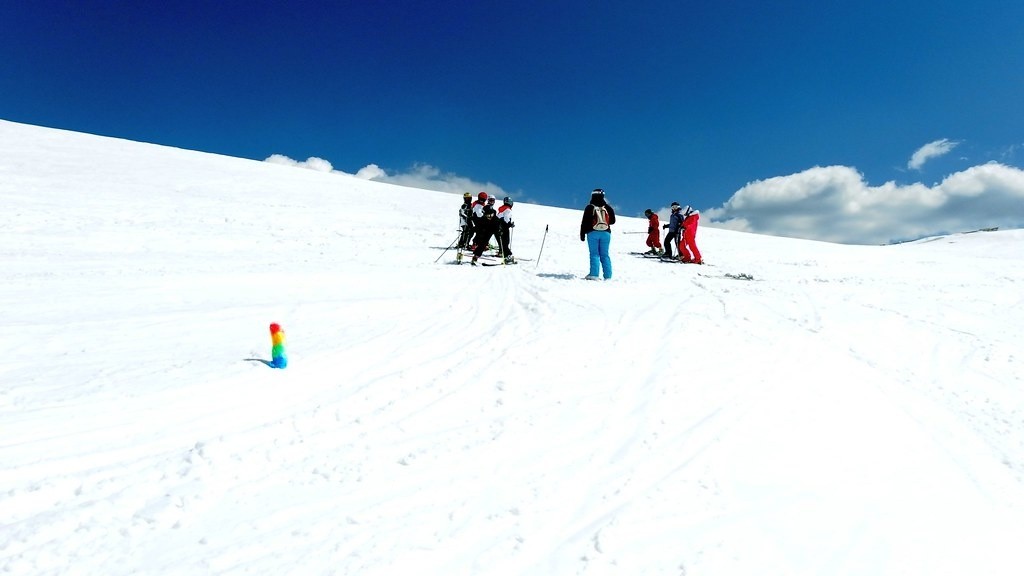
678, 205, 702, 262
644, 209, 664, 256
580, 188, 615, 281
663, 202, 684, 262
456, 191, 517, 267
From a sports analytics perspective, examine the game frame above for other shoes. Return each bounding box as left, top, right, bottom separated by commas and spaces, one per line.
585, 274, 599, 280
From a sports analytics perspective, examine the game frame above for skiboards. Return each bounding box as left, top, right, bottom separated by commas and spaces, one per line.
631, 249, 705, 265
430, 243, 533, 266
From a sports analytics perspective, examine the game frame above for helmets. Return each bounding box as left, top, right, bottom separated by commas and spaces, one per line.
591, 188, 606, 195
463, 193, 472, 197
504, 196, 513, 206
488, 195, 496, 201
478, 192, 487, 199
684, 205, 693, 215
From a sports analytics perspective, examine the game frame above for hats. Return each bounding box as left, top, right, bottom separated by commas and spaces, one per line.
671, 202, 679, 207
645, 209, 651, 214
